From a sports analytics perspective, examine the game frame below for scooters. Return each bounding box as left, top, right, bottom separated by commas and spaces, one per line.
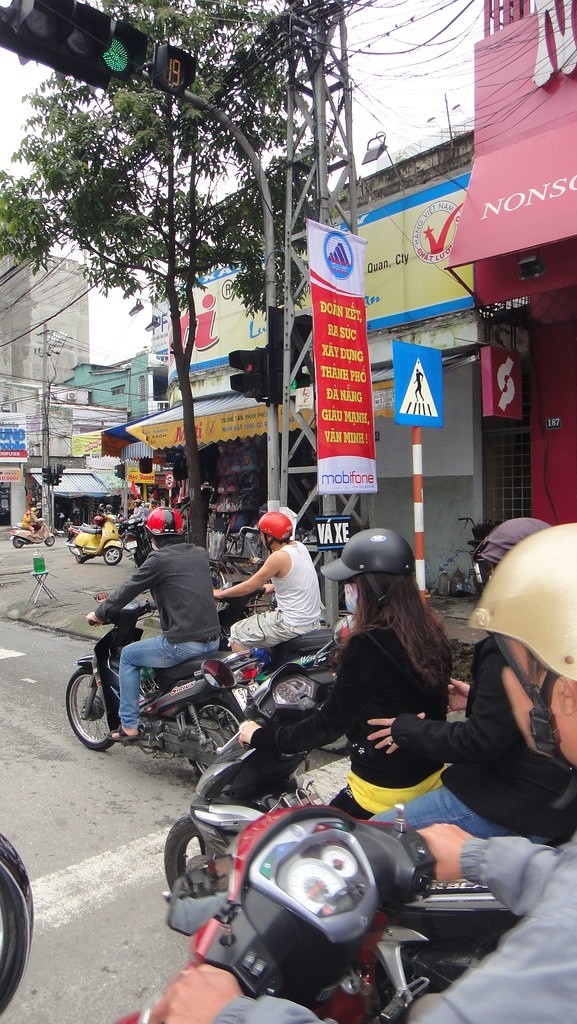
164, 618, 523, 965
116, 803, 454, 1024
9, 519, 55, 549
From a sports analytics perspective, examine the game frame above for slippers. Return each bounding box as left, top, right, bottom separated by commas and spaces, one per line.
105, 724, 139, 740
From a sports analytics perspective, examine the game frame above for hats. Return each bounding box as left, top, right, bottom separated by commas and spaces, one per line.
472, 517, 552, 563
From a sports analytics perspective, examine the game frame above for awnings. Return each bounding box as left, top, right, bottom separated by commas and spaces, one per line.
32, 471, 141, 497
100, 366, 393, 466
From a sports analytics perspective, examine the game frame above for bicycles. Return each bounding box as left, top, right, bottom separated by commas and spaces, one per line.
208, 515, 277, 615
458, 517, 501, 594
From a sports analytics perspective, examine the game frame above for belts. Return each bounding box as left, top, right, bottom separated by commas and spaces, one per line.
195, 634, 219, 644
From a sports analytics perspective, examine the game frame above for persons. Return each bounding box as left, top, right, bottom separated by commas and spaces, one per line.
95, 490, 189, 531
214, 511, 322, 686
148, 523, 577, 1024
302, 449, 323, 515
86, 508, 221, 739
21, 506, 44, 538
236, 527, 453, 820
54, 504, 64, 531
365, 517, 577, 847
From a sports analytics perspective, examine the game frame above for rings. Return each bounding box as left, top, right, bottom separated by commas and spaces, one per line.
388, 719, 390, 726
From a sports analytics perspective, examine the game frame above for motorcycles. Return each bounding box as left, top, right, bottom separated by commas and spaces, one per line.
65, 598, 267, 780
65, 504, 152, 570
212, 581, 351, 670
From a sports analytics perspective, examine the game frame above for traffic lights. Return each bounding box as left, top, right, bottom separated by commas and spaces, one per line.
228, 348, 266, 398
152, 41, 197, 95
0, 0, 147, 88
57, 465, 62, 485
42, 468, 51, 484
51, 473, 57, 485
268, 308, 314, 403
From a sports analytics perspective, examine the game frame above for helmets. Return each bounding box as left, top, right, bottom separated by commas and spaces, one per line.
320, 528, 416, 581
99, 503, 104, 510
145, 507, 184, 535
30, 507, 37, 512
106, 505, 114, 511
94, 516, 103, 526
133, 499, 141, 507
257, 511, 293, 543
469, 522, 575, 683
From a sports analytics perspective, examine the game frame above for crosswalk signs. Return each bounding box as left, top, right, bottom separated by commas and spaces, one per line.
392, 340, 443, 428
